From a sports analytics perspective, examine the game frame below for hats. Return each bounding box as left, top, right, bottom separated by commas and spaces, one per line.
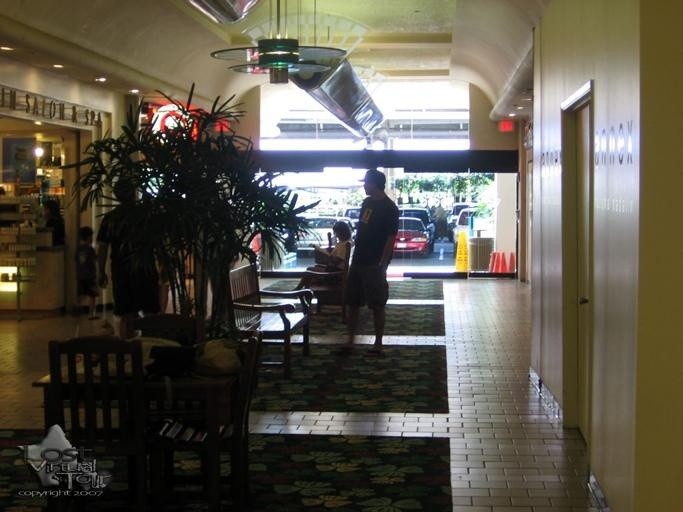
359, 170, 386, 189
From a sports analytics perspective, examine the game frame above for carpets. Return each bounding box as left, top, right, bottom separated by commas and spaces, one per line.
0, 281, 453, 512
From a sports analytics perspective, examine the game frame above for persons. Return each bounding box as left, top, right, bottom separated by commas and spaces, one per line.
76, 178, 169, 340
43, 200, 66, 246
292, 170, 399, 351
433, 204, 450, 242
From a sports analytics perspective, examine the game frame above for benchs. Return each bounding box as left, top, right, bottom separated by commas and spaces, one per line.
225, 240, 352, 367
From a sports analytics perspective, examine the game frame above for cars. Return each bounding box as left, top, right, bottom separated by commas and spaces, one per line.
446, 202, 480, 252
292, 203, 437, 258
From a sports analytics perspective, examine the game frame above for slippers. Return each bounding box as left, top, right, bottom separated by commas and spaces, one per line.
330, 346, 356, 355
361, 346, 385, 357
